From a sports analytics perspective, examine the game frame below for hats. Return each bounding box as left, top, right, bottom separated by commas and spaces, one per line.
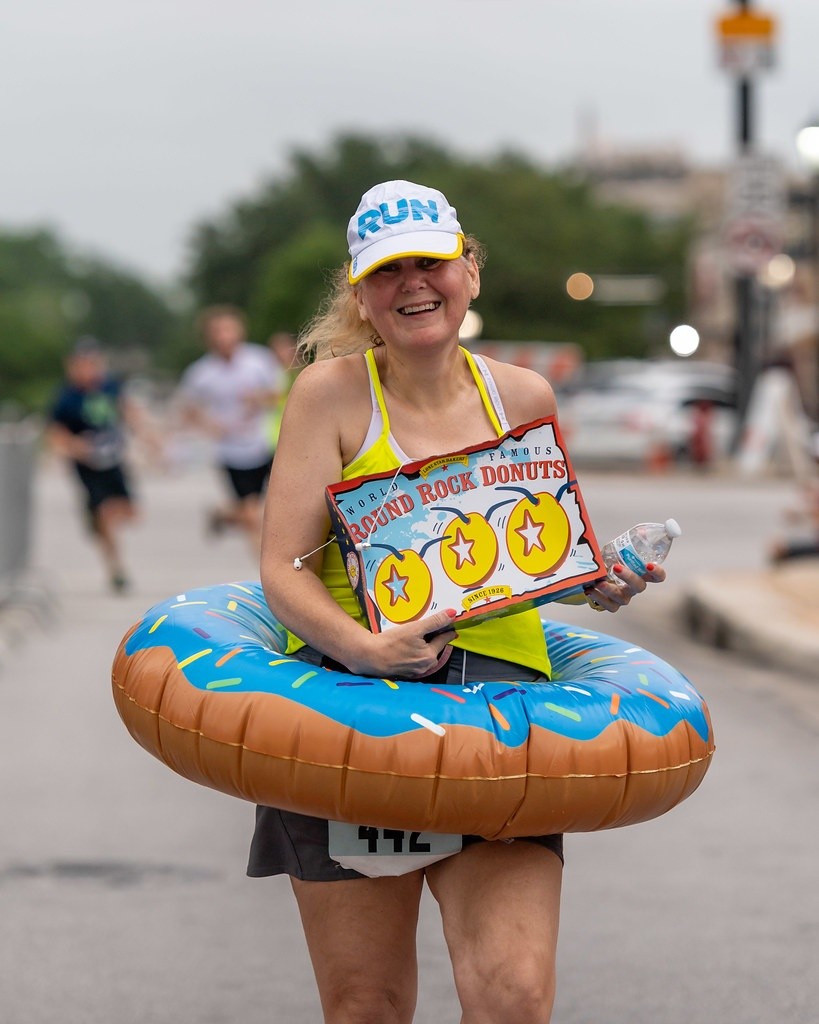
346, 180, 466, 285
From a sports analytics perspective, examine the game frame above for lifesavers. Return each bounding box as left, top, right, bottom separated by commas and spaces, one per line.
108, 570, 717, 839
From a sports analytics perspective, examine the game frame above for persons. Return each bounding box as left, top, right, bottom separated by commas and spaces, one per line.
174, 308, 311, 555
47, 341, 170, 593
245, 180, 666, 1024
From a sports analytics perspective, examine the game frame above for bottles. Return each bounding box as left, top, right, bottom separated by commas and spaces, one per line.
601, 519, 681, 585
